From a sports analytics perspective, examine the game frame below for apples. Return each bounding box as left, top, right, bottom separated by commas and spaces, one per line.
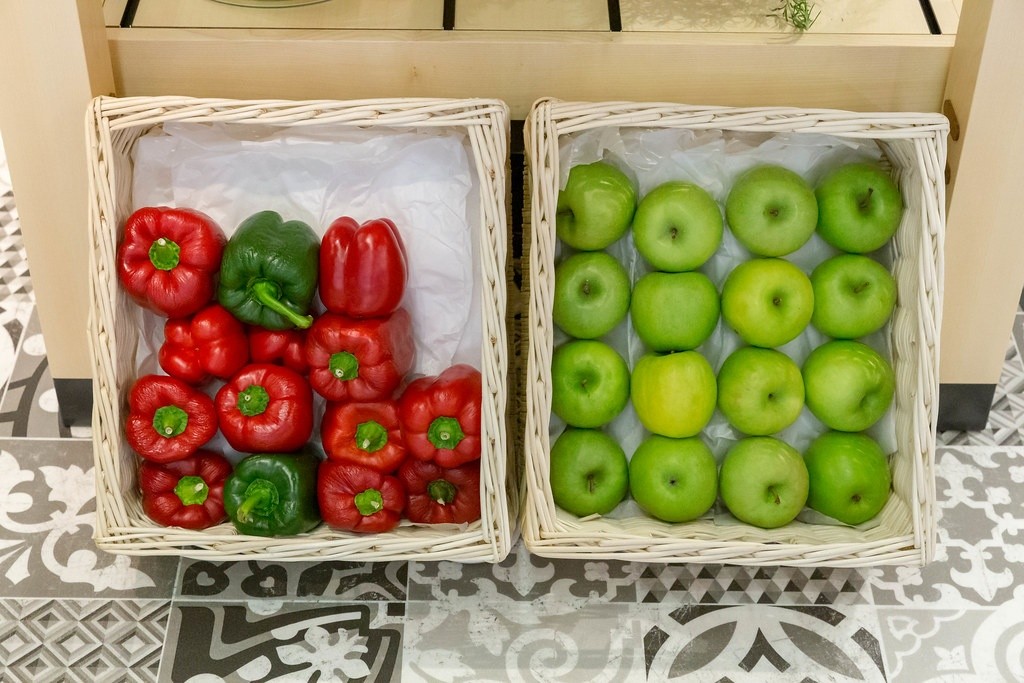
550, 161, 905, 527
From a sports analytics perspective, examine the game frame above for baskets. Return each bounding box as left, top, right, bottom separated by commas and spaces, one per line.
521, 97, 950, 568
83, 95, 520, 564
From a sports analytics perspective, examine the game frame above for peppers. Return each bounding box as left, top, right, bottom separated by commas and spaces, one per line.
116, 204, 484, 538
